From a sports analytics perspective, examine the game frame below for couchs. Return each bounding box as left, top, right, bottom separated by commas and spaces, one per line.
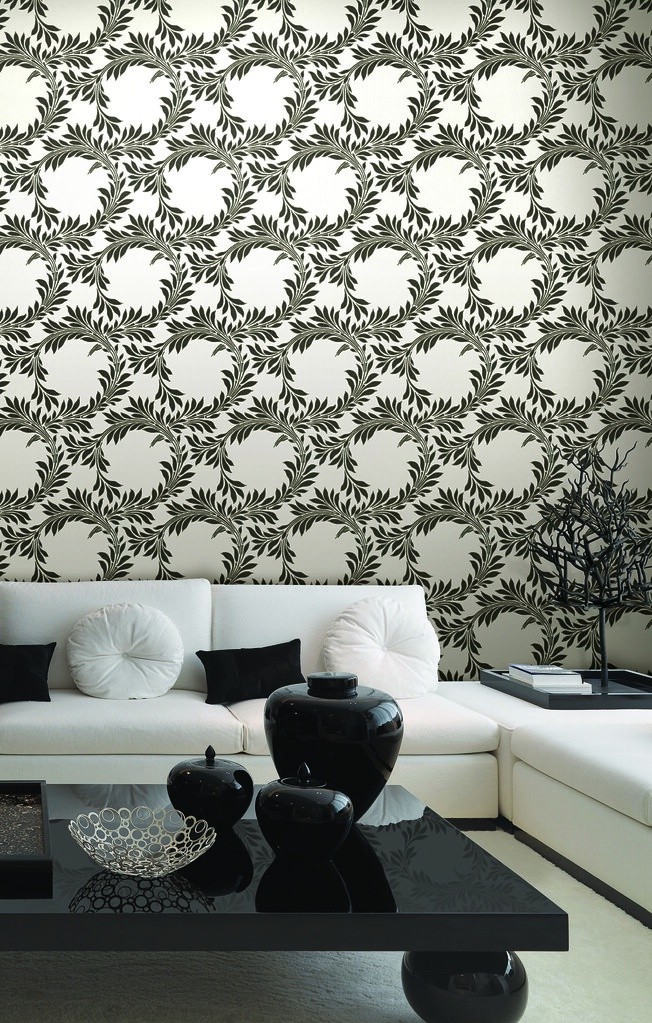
0, 578, 652, 930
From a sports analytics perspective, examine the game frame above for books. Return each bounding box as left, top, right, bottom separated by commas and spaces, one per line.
508, 663, 583, 687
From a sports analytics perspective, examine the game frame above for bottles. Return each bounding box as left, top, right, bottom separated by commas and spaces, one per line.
167, 744, 253, 832
255, 762, 353, 862
264, 669, 404, 830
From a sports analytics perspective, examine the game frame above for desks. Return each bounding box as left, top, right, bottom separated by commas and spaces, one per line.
0, 779, 569, 1022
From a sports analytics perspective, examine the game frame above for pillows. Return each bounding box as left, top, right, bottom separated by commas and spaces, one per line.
66, 602, 184, 700
323, 595, 440, 698
0, 641, 57, 705
195, 639, 307, 705
